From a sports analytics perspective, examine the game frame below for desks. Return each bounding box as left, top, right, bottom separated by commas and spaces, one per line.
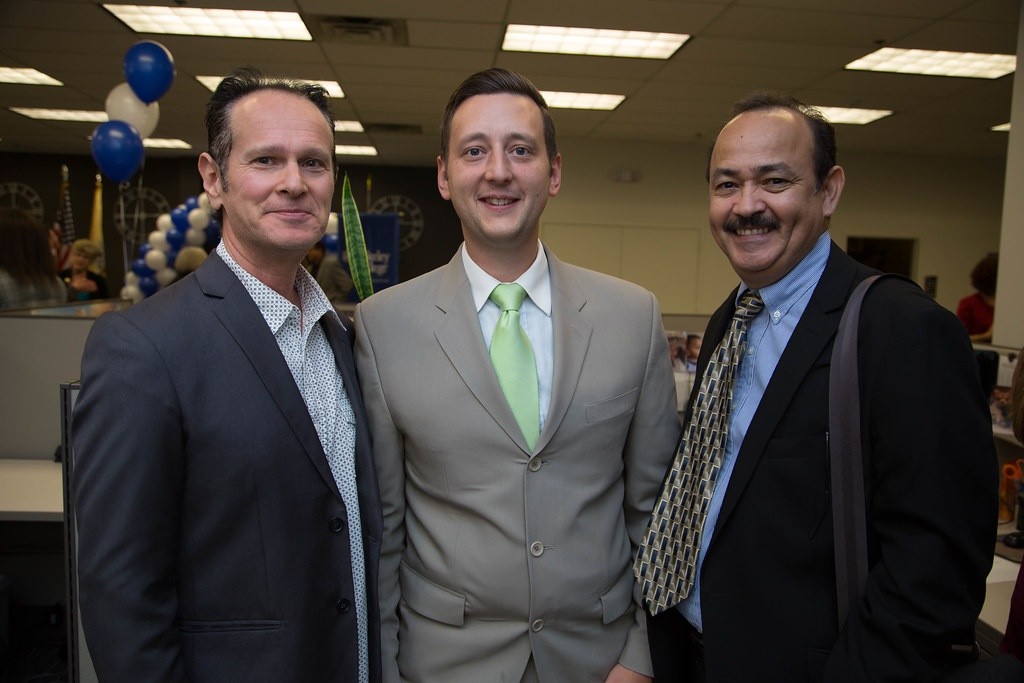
0, 457, 64, 554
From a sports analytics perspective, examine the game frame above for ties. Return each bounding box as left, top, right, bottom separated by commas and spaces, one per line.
632, 290, 765, 617
488, 283, 541, 453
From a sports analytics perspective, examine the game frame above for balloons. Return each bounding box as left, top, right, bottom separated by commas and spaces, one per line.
91, 121, 144, 183
124, 40, 176, 106
106, 82, 160, 140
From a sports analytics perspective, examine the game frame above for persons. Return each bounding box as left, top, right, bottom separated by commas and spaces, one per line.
665, 334, 702, 373
0, 208, 107, 312
302, 240, 354, 303
121, 192, 222, 304
350, 69, 682, 682
72, 66, 382, 683
634, 92, 999, 683
957, 253, 999, 346
